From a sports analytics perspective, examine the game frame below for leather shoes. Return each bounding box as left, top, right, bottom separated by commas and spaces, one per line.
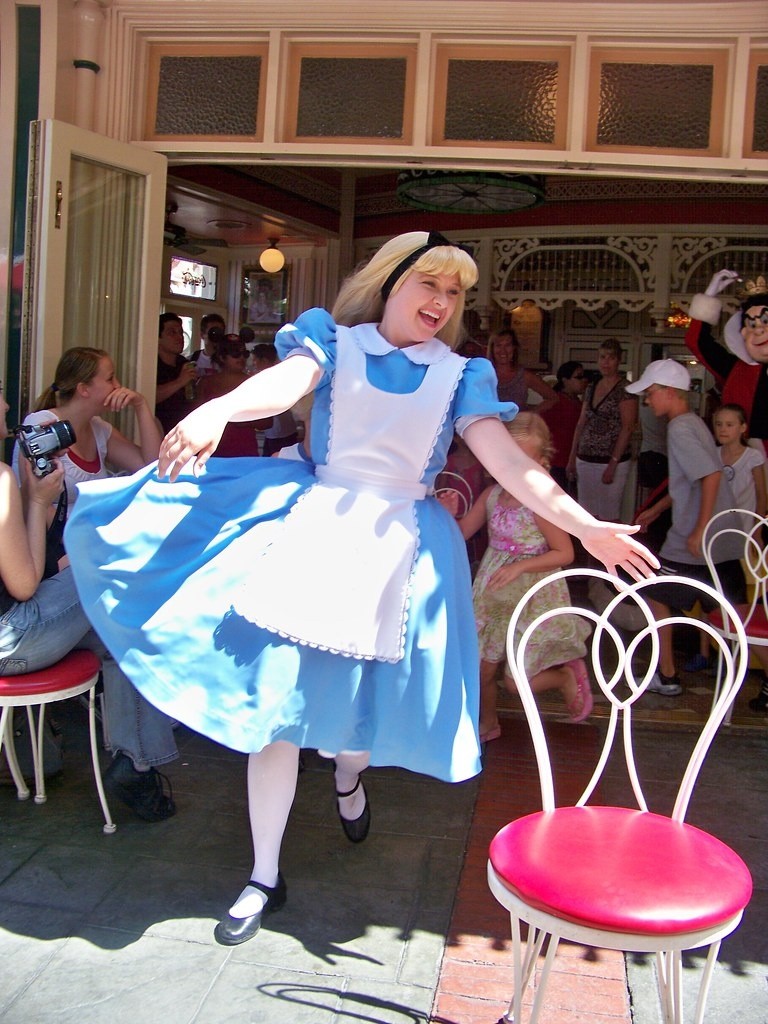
332, 760, 371, 843
216, 870, 286, 945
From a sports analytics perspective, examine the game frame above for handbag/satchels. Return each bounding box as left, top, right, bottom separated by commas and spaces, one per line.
0, 703, 64, 784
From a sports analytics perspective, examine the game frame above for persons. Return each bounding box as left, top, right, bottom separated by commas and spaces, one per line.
0, 346, 179, 824
152, 307, 768, 745
64, 232, 663, 945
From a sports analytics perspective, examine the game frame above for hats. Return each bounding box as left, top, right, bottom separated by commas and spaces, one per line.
624, 358, 691, 397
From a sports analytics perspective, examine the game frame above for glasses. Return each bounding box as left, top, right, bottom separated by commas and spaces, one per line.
218, 350, 250, 359
570, 373, 587, 381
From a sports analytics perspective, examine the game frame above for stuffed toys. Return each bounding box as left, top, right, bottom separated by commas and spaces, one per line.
684, 269, 768, 603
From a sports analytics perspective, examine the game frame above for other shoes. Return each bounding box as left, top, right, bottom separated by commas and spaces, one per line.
748, 692, 768, 710
682, 653, 713, 672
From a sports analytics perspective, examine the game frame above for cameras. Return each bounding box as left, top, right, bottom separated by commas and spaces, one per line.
14, 420, 77, 478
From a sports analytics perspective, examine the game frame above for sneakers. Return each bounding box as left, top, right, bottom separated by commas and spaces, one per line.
102, 749, 176, 823
622, 666, 683, 697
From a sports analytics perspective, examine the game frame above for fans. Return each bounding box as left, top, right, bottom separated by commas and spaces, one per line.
163, 201, 230, 256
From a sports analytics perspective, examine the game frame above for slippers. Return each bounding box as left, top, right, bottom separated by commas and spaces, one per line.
478, 724, 501, 744
563, 658, 594, 722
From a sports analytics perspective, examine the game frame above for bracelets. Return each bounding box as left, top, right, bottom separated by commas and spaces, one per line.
612, 455, 619, 462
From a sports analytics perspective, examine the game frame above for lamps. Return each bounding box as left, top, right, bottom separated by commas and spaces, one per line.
395, 168, 546, 215
259, 238, 284, 274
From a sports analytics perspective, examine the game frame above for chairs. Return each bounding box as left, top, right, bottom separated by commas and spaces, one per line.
701, 508, 767, 727
487, 568, 753, 1024
0, 645, 118, 833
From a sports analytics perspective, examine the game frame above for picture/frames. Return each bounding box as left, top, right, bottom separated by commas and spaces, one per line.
239, 264, 294, 332
